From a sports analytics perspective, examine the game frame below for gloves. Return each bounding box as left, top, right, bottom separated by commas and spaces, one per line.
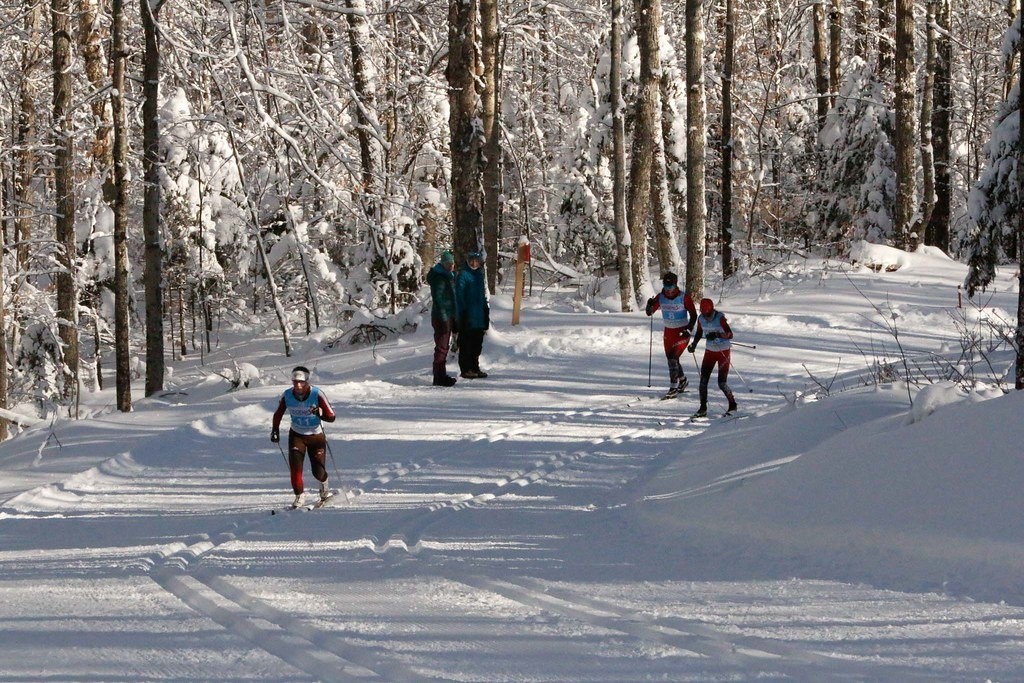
681, 327, 692, 338
688, 342, 697, 352
441, 322, 450, 333
706, 332, 720, 340
646, 298, 654, 310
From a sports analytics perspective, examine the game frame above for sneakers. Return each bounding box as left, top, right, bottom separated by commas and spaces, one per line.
665, 388, 678, 397
320, 478, 328, 498
677, 377, 688, 392
293, 492, 305, 507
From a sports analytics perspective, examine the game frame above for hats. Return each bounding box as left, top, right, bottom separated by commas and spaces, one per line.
291, 371, 310, 381
441, 251, 454, 263
664, 272, 677, 285
700, 299, 713, 314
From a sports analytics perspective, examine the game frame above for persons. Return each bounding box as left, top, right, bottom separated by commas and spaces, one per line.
270, 367, 335, 509
456, 252, 489, 378
426, 251, 456, 386
647, 273, 697, 401
688, 297, 739, 422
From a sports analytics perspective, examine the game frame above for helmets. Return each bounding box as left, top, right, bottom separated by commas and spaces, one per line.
467, 251, 482, 260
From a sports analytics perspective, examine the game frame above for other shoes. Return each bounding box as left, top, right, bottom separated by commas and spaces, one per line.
433, 375, 457, 386
473, 369, 487, 377
462, 371, 478, 378
694, 410, 707, 417
725, 403, 737, 417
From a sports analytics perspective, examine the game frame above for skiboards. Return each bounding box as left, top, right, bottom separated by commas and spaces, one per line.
627, 389, 690, 408
272, 492, 338, 515
658, 410, 740, 426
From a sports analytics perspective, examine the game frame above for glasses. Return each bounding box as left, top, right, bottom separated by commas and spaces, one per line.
703, 313, 713, 317
665, 285, 675, 289
